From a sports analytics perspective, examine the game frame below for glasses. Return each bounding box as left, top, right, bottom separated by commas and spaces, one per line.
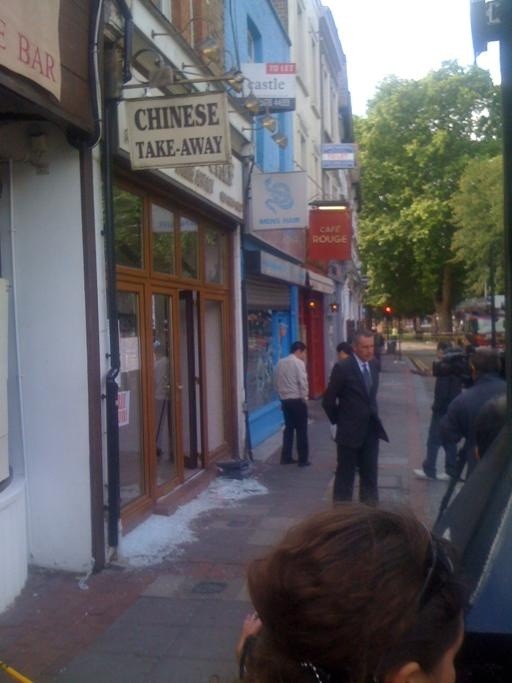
415, 521, 450, 617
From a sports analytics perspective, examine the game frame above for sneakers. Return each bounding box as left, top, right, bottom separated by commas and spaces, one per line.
281, 459, 311, 466
411, 467, 453, 482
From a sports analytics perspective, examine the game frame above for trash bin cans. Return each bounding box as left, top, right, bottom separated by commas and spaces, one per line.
387, 341, 396, 353
498, 352, 504, 376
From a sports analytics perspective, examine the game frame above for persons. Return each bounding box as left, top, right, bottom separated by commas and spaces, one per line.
151, 323, 177, 460
460, 334, 478, 389
473, 395, 507, 462
327, 341, 350, 442
233, 499, 467, 683
407, 339, 464, 481
371, 327, 384, 369
322, 328, 390, 508
276, 339, 311, 465
437, 347, 507, 472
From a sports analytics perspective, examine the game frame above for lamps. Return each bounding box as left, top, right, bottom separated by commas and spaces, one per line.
151, 15, 287, 146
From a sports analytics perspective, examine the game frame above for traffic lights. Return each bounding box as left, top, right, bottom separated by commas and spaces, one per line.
383, 302, 393, 321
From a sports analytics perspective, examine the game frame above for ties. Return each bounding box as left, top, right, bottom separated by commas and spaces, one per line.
361, 363, 372, 398
